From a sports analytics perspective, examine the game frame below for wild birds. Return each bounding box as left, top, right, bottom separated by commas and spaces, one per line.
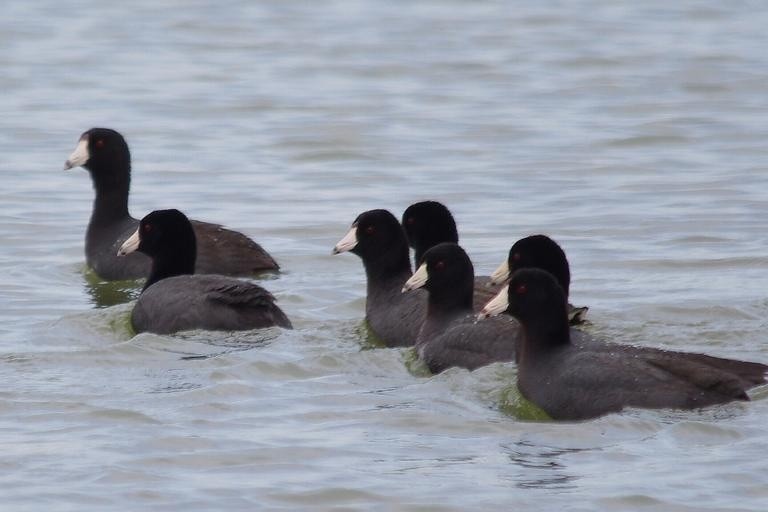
330, 208, 429, 349
399, 200, 588, 328
400, 242, 594, 375
61, 127, 280, 280
116, 208, 294, 336
475, 269, 768, 422
485, 234, 571, 305
